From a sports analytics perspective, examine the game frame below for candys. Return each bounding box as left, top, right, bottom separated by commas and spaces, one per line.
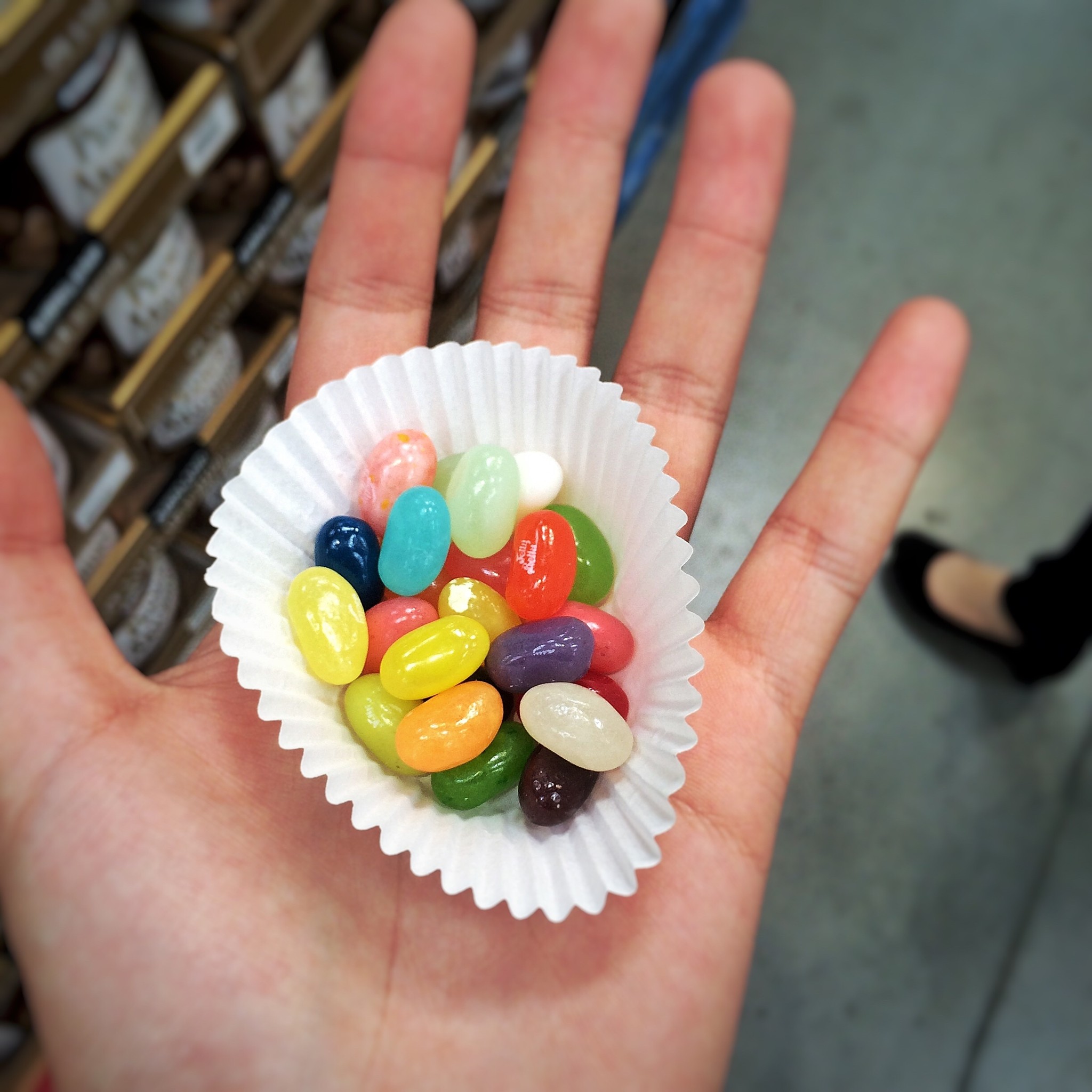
280, 427, 636, 826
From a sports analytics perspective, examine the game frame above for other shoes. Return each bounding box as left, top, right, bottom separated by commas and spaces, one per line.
891, 529, 1045, 689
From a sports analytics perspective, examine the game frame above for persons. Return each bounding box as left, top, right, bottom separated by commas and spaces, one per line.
887, 499, 1092, 685
1, 0, 971, 1091
